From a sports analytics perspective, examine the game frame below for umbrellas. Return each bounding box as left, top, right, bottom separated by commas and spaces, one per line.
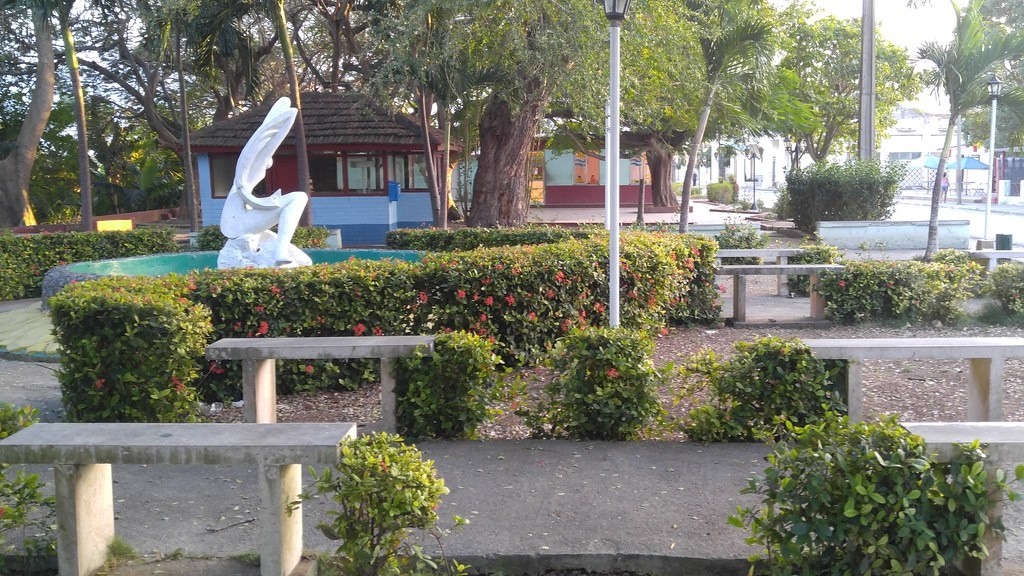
910, 151, 990, 196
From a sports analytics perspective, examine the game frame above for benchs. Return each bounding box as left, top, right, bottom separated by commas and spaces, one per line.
205, 335, 435, 435
900, 421, 1024, 576
929, 187, 985, 196
713, 265, 848, 321
962, 250, 1024, 272
0, 421, 354, 576
713, 248, 805, 296
800, 336, 1024, 425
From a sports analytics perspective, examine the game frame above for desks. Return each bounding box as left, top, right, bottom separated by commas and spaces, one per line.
922, 180, 975, 195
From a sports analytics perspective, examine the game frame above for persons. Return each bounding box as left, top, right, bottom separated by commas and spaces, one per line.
692, 171, 698, 186
575, 175, 584, 184
939, 172, 950, 204
590, 175, 597, 184
